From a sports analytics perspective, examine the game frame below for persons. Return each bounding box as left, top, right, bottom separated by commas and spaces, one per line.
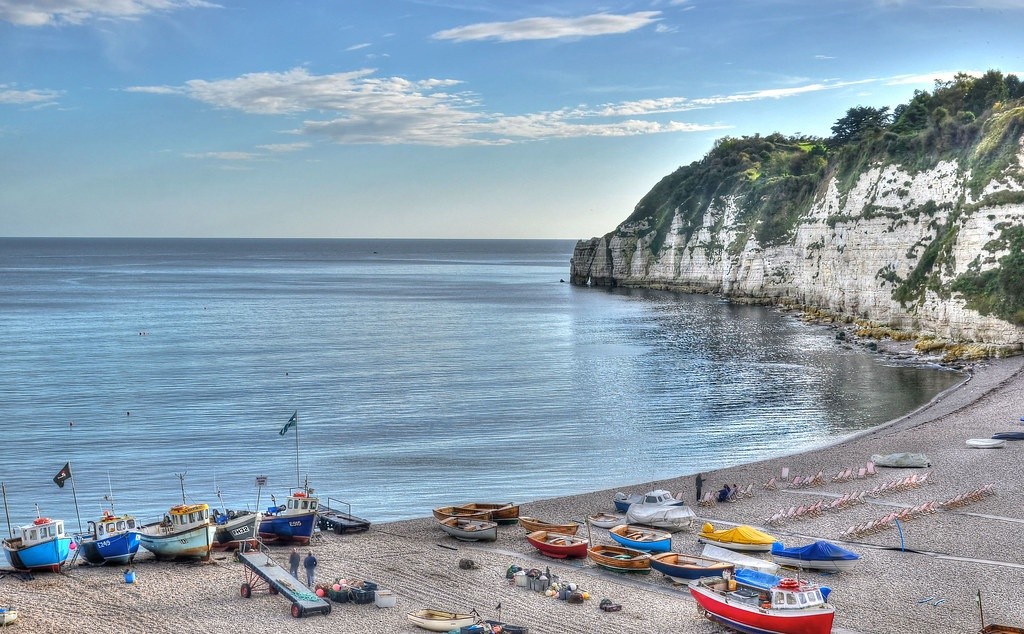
696, 473, 706, 501
718, 484, 738, 503
289, 548, 300, 581
165, 516, 173, 532
304, 551, 317, 588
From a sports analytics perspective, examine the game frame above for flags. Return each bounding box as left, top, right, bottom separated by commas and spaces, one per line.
279, 411, 297, 436
53, 462, 72, 488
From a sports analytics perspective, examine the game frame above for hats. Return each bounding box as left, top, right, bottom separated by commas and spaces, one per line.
308, 550, 314, 555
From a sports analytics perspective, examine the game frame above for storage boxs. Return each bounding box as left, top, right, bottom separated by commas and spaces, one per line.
315, 579, 396, 608
460, 620, 529, 634
515, 571, 549, 592
124, 571, 135, 582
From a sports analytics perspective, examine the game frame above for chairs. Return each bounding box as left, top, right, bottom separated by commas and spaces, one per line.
675, 462, 998, 542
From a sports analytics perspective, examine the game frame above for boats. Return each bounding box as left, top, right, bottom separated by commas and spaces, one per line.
613, 490, 685, 513
695, 523, 778, 553
768, 541, 861, 574
729, 568, 831, 603
1020, 416, 1024, 421
608, 524, 672, 554
717, 293, 730, 305
974, 588, 1024, 634
650, 552, 735, 585
0, 606, 19, 626
460, 620, 529, 634
626, 503, 697, 532
432, 491, 626, 558
966, 438, 1007, 449
587, 545, 651, 575
992, 431, 1024, 442
687, 564, 834, 634
1, 409, 320, 573
407, 608, 475, 631
871, 453, 929, 468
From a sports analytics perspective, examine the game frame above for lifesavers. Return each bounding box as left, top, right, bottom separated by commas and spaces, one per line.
34, 518, 48, 525
294, 492, 306, 497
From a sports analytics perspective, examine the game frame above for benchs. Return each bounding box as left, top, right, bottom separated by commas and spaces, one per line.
636, 534, 654, 541
675, 560, 696, 564
549, 537, 563, 544
626, 531, 639, 537
